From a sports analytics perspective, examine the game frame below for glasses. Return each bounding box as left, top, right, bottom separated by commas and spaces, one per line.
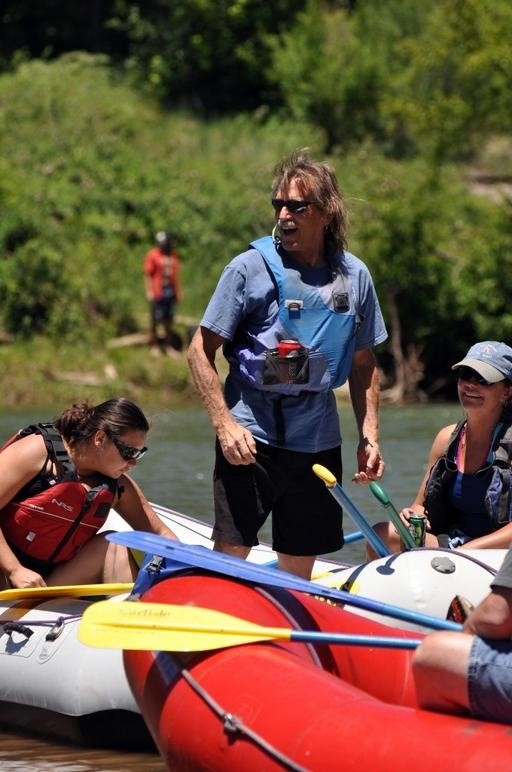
270, 198, 311, 215
114, 437, 149, 461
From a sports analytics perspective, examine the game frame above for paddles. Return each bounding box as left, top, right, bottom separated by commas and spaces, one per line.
105, 529, 466, 635
78, 601, 422, 652
0, 584, 136, 599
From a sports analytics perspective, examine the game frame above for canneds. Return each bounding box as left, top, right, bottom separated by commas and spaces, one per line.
279, 340, 301, 381
408, 515, 426, 548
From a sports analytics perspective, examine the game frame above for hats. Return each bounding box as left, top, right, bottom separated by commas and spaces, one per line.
451, 340, 512, 384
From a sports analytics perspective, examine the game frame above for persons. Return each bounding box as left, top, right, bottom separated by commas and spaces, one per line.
143, 231, 181, 358
410, 539, 512, 726
0, 397, 180, 601
186, 146, 389, 581
367, 341, 512, 560
